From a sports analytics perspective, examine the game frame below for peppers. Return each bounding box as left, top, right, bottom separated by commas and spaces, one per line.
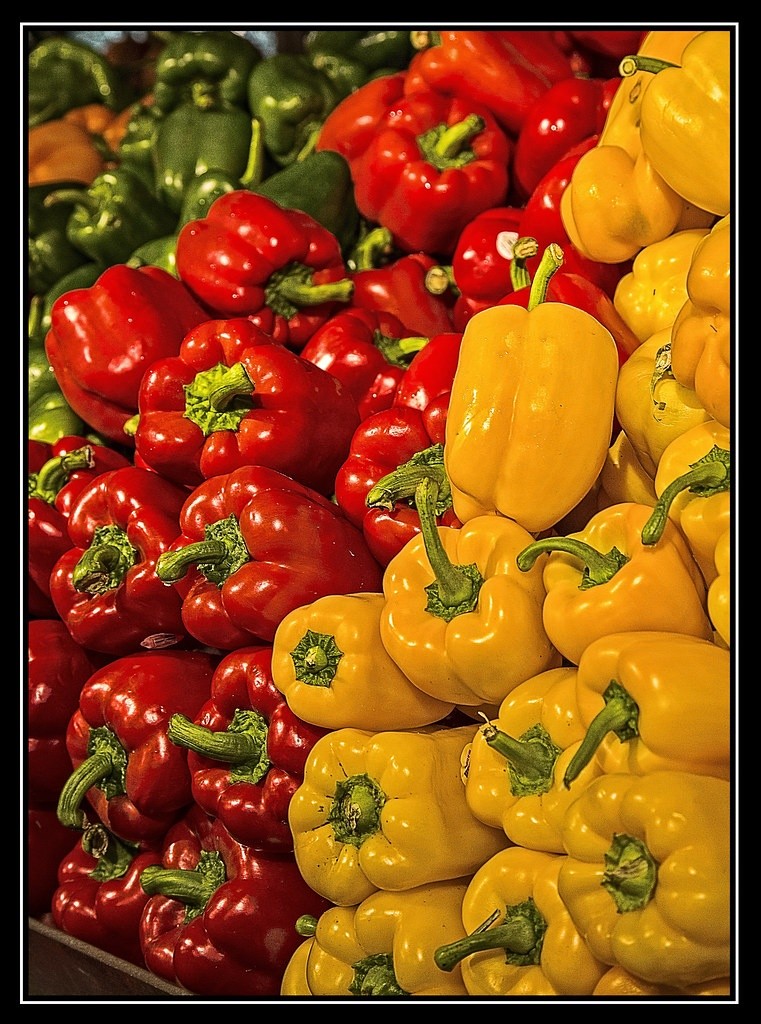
28, 31, 731, 996
